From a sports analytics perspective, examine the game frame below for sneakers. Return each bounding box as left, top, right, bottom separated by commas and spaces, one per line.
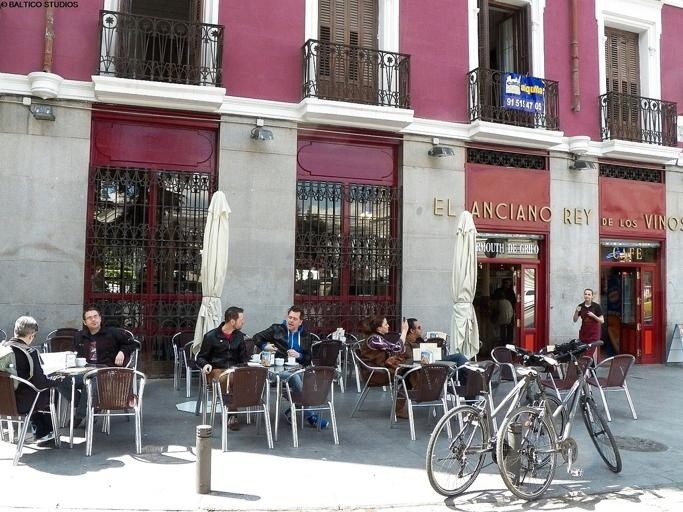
284, 408, 291, 424
309, 415, 330, 430
68, 415, 82, 429
227, 415, 239, 431
36, 432, 62, 447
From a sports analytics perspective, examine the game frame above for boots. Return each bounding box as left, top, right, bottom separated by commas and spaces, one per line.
396, 399, 408, 418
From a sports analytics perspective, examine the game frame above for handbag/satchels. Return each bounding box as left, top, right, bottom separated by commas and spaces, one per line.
385, 351, 406, 369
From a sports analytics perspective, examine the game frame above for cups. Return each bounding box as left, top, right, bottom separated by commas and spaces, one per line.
274, 358, 283, 365
287, 357, 295, 363
75, 357, 87, 367
274, 366, 283, 372
250, 353, 260, 361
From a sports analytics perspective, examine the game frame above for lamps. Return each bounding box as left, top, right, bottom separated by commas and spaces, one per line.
569, 160, 597, 171
249, 124, 274, 143
426, 142, 454, 162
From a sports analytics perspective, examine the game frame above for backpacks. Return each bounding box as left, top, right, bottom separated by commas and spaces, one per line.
0, 328, 34, 392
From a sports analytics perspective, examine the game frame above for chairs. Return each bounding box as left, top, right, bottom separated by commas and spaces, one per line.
0, 316, 146, 467
173, 329, 346, 454
353, 328, 640, 439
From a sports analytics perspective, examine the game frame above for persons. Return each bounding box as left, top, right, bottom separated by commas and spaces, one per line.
402, 317, 487, 418
572, 288, 605, 374
362, 312, 415, 420
57, 305, 137, 427
0, 315, 63, 441
196, 306, 250, 430
253, 305, 330, 428
493, 278, 517, 342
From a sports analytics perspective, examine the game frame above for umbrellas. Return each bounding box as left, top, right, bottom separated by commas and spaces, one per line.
448, 210, 481, 358
189, 190, 232, 364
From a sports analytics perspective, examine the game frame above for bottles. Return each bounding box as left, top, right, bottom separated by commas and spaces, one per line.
260, 348, 275, 366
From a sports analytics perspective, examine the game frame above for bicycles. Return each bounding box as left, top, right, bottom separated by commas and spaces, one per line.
495, 339, 623, 501
423, 342, 567, 496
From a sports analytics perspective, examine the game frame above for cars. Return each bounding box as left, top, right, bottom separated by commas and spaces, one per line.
523, 289, 534, 311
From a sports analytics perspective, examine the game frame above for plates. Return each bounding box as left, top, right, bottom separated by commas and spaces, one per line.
248, 359, 260, 363
284, 361, 298, 366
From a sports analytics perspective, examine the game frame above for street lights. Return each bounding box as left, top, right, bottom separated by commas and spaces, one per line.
361, 211, 373, 299
